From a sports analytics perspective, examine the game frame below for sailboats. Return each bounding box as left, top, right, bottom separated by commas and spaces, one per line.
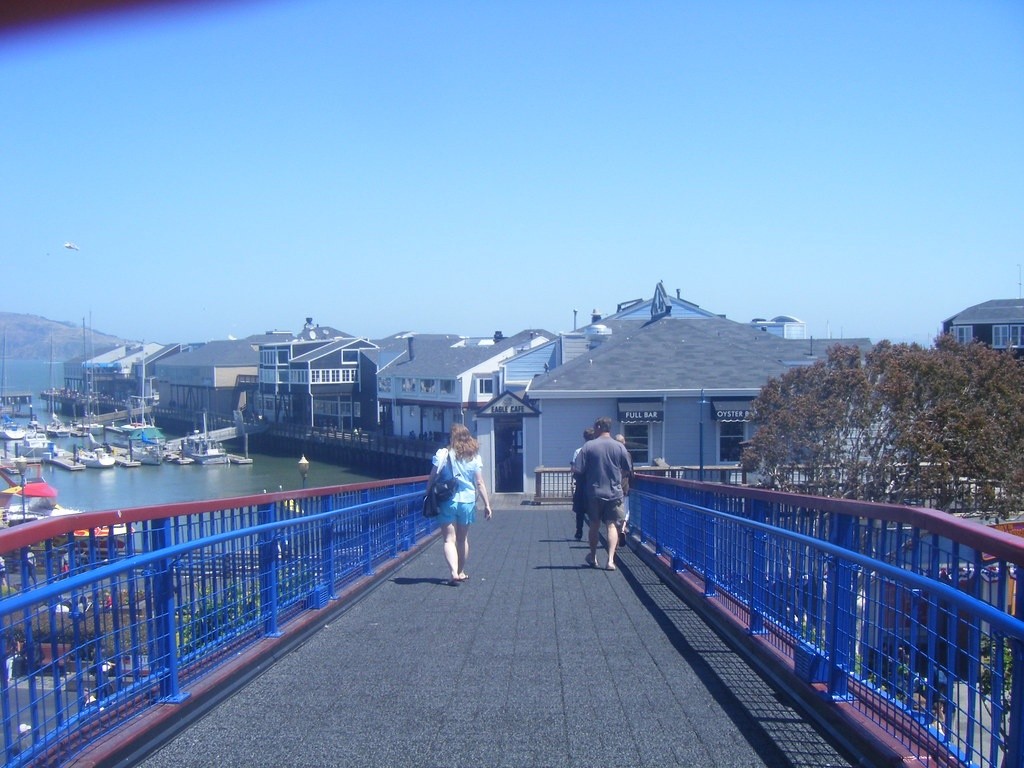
41, 318, 105, 437
71, 311, 115, 468
104, 355, 166, 445
0, 335, 27, 440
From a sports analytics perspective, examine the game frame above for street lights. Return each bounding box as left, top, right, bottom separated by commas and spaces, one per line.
298, 453, 310, 489
696, 388, 711, 481
15, 454, 30, 592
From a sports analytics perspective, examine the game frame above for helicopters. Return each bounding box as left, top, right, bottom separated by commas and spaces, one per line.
64, 242, 78, 250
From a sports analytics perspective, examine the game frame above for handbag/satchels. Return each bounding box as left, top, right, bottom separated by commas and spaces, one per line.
431, 453, 458, 498
422, 474, 440, 518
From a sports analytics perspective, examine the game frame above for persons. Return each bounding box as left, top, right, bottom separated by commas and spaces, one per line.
5, 634, 43, 682
89, 648, 116, 708
932, 701, 945, 736
872, 642, 887, 677
84, 687, 105, 713
27, 544, 38, 589
61, 550, 69, 572
570, 416, 631, 570
0, 556, 7, 586
12, 724, 32, 757
408, 430, 432, 442
353, 426, 363, 440
34, 595, 88, 614
169, 400, 176, 410
426, 423, 492, 584
99, 591, 112, 614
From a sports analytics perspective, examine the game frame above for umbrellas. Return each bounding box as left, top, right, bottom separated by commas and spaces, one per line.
1, 483, 57, 510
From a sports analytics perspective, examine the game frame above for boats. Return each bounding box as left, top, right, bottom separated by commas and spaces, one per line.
16, 422, 51, 456
129, 432, 195, 466
181, 411, 230, 465
0, 456, 134, 550
102, 441, 141, 466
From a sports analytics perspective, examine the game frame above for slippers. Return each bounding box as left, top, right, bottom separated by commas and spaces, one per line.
606, 562, 616, 570
585, 553, 599, 567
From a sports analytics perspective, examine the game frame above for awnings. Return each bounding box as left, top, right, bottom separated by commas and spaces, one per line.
711, 397, 755, 422
617, 398, 663, 425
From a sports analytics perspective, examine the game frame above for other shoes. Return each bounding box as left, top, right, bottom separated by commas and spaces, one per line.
575, 530, 583, 541
